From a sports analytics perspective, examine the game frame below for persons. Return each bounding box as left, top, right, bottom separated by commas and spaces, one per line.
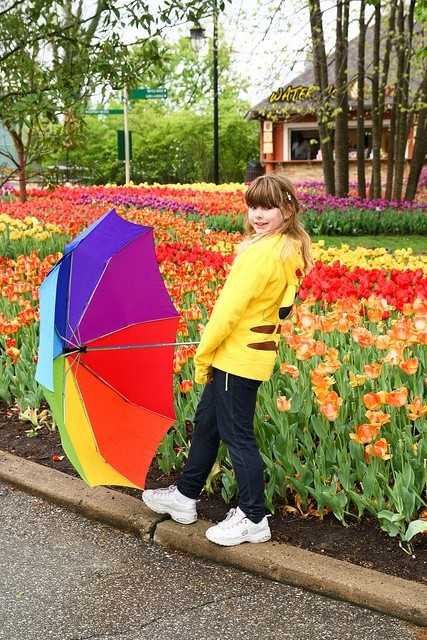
142, 172, 309, 549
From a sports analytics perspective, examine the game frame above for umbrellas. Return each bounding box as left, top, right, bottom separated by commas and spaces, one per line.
34, 207, 201, 492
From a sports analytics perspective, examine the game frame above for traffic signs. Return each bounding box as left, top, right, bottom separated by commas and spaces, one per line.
126, 87, 167, 99
84, 109, 123, 114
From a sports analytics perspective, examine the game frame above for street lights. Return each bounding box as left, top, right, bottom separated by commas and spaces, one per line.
189, 27, 218, 185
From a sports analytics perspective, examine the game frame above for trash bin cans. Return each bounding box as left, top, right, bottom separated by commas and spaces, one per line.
246, 162, 264, 182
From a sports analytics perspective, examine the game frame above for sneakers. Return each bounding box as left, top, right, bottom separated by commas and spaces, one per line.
141, 485, 201, 524
204, 506, 272, 546
